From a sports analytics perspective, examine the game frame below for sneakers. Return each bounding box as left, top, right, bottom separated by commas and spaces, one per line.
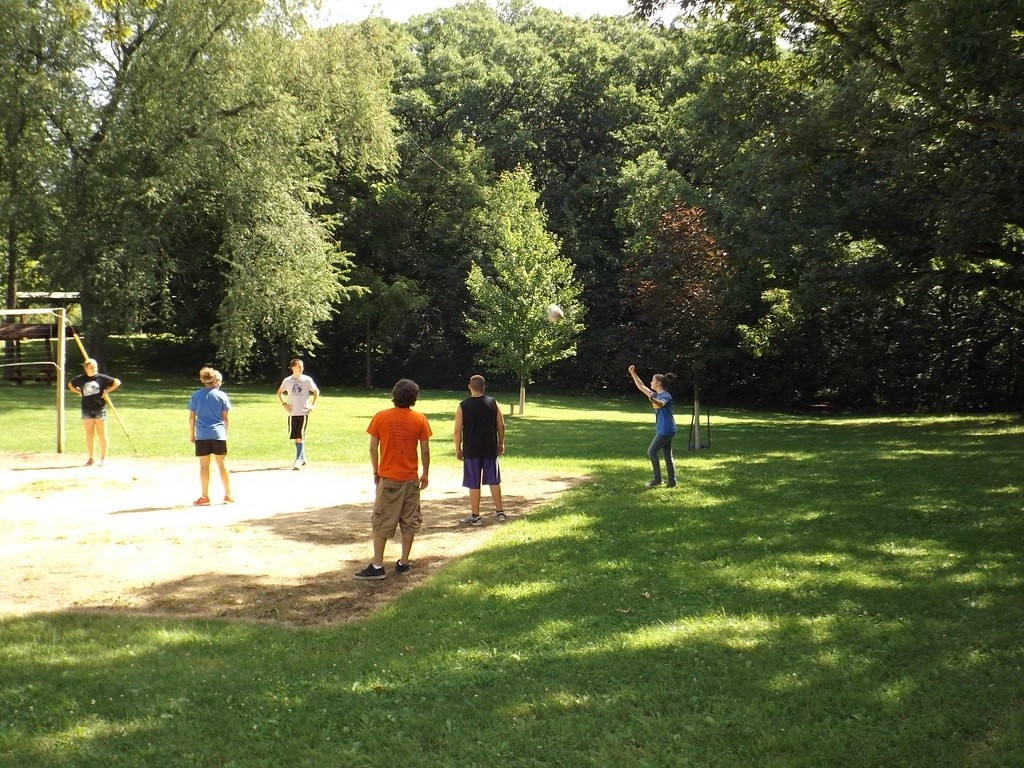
460, 516, 483, 526
193, 497, 210, 506
397, 559, 410, 575
352, 563, 385, 580
223, 496, 234, 503
496, 513, 506, 522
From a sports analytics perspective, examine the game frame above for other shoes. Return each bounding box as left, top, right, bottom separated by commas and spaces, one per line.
98, 459, 106, 465
293, 460, 301, 470
302, 460, 306, 465
645, 479, 663, 488
84, 458, 94, 466
665, 482, 676, 488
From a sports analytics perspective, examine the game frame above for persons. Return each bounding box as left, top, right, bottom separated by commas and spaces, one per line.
454, 375, 506, 527
187, 367, 236, 505
353, 379, 433, 579
68, 358, 122, 467
277, 359, 320, 470
629, 365, 677, 488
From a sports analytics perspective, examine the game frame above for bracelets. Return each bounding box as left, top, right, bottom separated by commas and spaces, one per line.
373, 473, 378, 475
282, 402, 287, 405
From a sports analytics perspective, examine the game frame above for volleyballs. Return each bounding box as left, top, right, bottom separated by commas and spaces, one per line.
547, 304, 564, 323
